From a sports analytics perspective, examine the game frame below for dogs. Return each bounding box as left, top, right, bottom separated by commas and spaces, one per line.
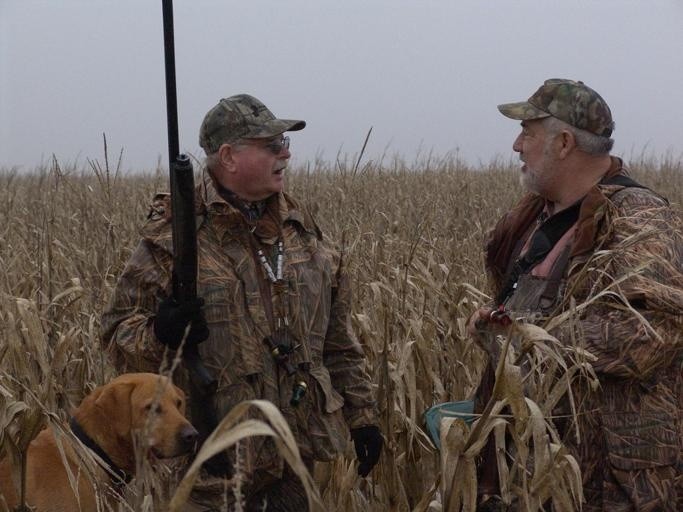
0, 373, 201, 512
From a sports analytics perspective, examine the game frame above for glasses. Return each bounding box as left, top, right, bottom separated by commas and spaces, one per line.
233, 136, 289, 153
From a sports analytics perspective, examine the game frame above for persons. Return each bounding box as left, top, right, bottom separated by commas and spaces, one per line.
466, 78, 682, 512
98, 93, 383, 512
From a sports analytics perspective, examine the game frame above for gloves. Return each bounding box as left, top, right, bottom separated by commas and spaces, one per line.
351, 426, 383, 477
155, 293, 210, 349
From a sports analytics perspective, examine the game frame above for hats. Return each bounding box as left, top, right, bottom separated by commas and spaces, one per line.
498, 80, 613, 136
199, 94, 306, 155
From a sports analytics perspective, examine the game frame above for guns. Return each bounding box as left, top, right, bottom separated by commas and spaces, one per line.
154, 0, 238, 478
